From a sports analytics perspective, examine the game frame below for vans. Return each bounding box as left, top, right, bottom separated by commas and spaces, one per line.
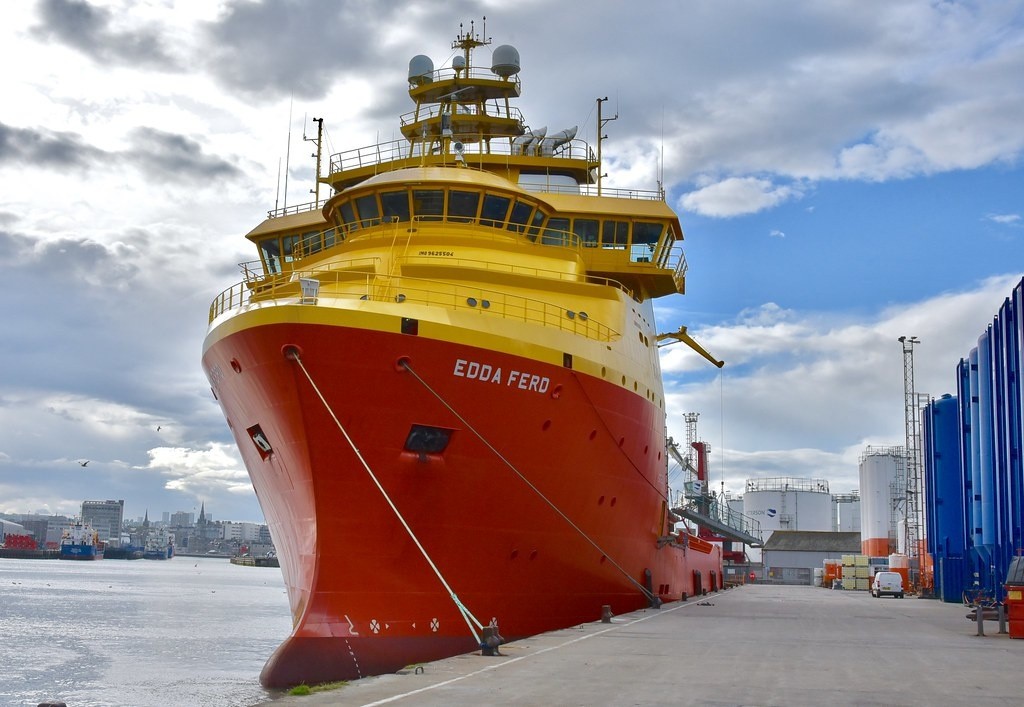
871, 572, 904, 598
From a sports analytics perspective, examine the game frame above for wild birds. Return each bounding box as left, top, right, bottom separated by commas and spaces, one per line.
156, 426, 162, 432
78, 461, 90, 466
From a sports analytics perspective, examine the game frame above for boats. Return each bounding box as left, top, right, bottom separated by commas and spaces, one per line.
199, 14, 723, 694
59, 518, 104, 560
104, 527, 175, 561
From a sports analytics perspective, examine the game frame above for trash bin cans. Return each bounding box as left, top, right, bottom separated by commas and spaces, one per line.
1004, 584, 1024, 640
